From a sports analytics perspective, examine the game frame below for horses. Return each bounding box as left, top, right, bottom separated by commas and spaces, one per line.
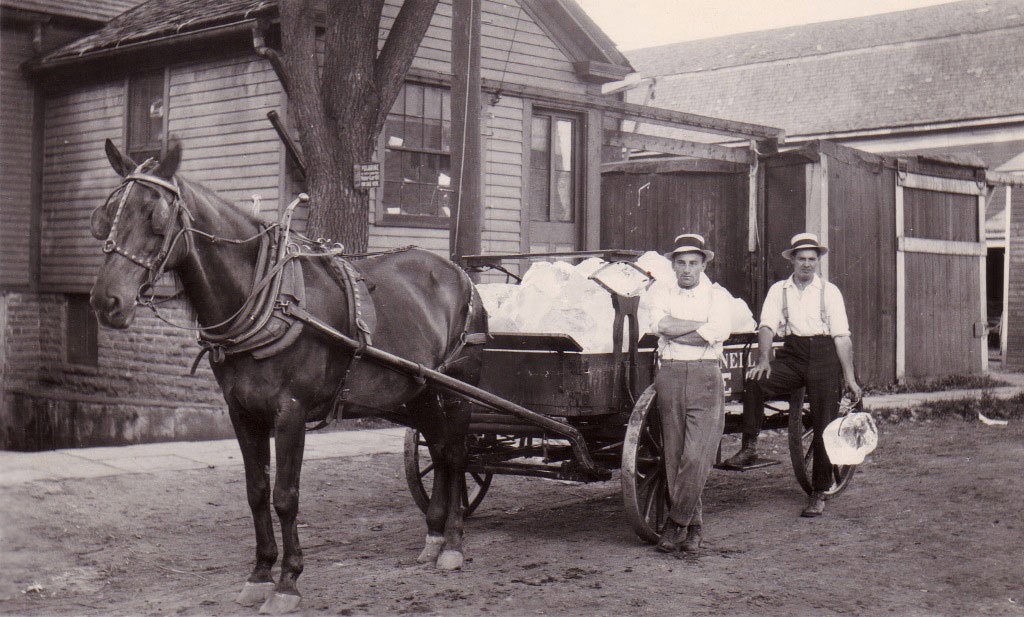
87, 139, 488, 613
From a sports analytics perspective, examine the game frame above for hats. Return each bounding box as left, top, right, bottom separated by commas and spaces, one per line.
664, 234, 715, 262
781, 233, 828, 261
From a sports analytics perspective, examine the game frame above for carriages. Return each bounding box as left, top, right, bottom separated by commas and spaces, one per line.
88, 136, 865, 617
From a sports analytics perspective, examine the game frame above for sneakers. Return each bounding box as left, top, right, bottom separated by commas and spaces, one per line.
724, 447, 757, 468
801, 491, 829, 517
680, 525, 702, 553
656, 516, 686, 553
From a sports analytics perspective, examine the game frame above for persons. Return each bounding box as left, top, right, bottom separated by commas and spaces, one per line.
648, 233, 733, 554
722, 233, 862, 518
